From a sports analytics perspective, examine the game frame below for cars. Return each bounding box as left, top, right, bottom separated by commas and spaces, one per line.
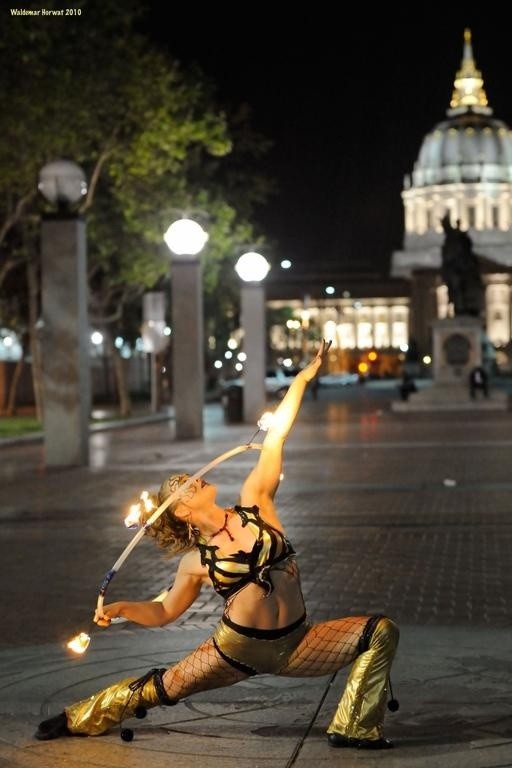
221, 363, 301, 400
320, 370, 360, 387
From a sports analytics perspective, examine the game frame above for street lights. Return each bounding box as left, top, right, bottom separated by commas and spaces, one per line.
159, 217, 213, 440
31, 159, 97, 468
233, 250, 272, 424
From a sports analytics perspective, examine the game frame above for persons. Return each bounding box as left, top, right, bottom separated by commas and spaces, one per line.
33, 334, 406, 753
310, 373, 320, 401
393, 372, 416, 400
469, 366, 492, 401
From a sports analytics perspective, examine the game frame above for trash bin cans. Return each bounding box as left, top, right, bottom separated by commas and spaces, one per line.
219, 385, 242, 423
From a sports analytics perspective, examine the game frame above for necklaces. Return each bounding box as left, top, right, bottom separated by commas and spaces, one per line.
204, 511, 238, 544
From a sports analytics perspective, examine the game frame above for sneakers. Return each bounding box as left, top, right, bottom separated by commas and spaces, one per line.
34, 711, 90, 741
328, 733, 395, 750
